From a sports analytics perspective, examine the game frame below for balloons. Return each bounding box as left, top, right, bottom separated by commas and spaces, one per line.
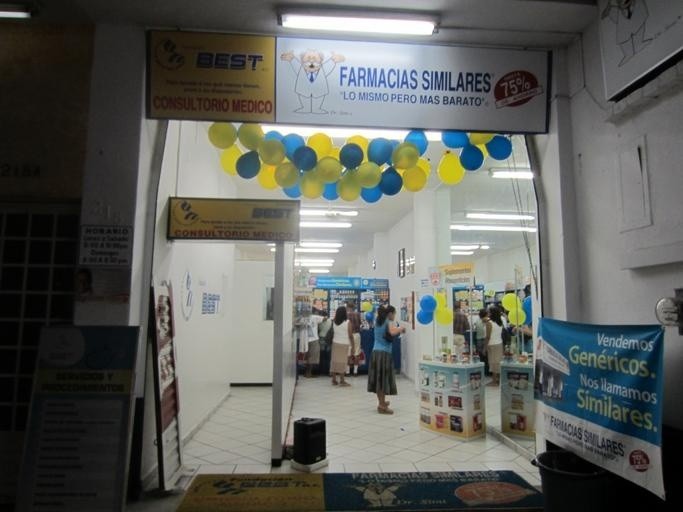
394, 130, 431, 192
438, 153, 464, 185
258, 130, 341, 200
501, 292, 532, 328
441, 130, 512, 170
416, 293, 453, 327
336, 136, 402, 202
211, 121, 264, 178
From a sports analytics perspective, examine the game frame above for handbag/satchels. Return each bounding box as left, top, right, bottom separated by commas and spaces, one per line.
500, 328, 510, 344
324, 328, 334, 345
383, 330, 392, 342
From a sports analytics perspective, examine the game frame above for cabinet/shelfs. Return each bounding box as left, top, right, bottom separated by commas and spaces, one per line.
312, 287, 391, 371
452, 285, 487, 355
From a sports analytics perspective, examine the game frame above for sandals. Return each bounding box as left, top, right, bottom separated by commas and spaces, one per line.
378, 400, 393, 414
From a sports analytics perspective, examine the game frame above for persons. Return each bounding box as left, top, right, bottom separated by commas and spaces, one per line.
76, 269, 105, 297
368, 305, 407, 414
295, 297, 389, 385
475, 301, 533, 386
453, 302, 470, 356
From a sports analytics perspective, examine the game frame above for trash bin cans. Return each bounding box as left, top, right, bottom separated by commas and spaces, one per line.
531, 450, 617, 512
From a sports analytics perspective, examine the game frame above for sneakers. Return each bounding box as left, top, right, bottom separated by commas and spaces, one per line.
338, 383, 351, 386
332, 381, 338, 385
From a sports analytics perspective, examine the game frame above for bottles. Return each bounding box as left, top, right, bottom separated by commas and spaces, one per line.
339, 290, 355, 298
450, 353, 456, 364
462, 342, 469, 363
442, 352, 448, 362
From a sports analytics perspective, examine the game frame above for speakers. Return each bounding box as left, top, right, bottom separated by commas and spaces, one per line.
294, 418, 326, 465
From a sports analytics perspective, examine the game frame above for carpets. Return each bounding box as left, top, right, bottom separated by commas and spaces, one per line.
177, 469, 544, 512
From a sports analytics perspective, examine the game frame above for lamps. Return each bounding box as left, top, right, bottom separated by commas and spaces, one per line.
274, 5, 441, 38
